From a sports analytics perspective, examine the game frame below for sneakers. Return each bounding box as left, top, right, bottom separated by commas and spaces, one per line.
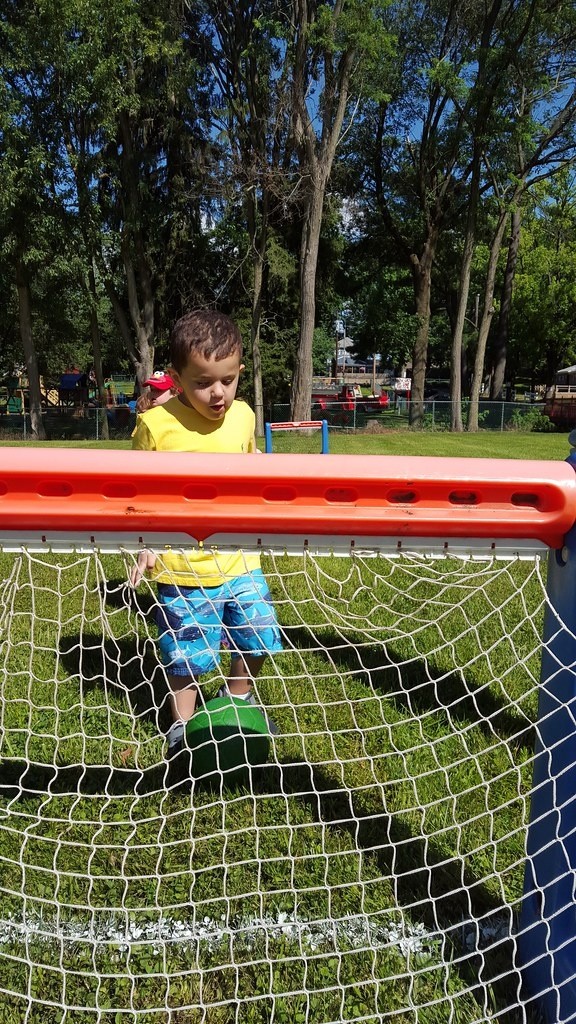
216, 685, 279, 735
167, 724, 187, 753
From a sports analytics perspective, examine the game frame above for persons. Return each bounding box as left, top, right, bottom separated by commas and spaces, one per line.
130, 310, 284, 754
130, 369, 185, 437
128, 393, 138, 434
328, 386, 355, 430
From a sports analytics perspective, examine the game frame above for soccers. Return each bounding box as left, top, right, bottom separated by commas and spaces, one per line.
180, 695, 272, 791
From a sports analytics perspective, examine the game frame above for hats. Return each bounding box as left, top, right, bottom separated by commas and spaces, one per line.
142, 370, 184, 393
